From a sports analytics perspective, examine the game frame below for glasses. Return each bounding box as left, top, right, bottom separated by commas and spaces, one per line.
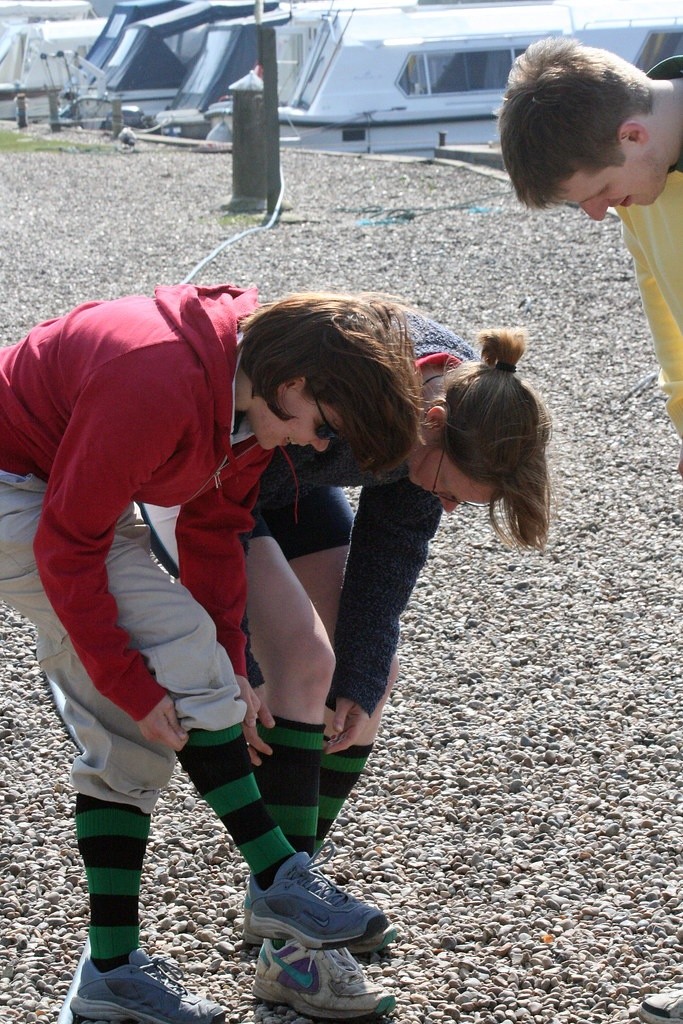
306, 388, 342, 439
429, 444, 492, 508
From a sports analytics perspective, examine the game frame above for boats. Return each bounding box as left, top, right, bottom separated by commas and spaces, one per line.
0, 0, 682, 173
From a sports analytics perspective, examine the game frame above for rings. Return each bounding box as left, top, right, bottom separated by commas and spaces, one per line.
247, 742, 250, 747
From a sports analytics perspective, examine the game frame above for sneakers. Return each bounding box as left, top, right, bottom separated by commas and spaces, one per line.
639, 990, 683, 1024
252, 937, 394, 1019
242, 840, 391, 950
245, 882, 397, 954
69, 947, 225, 1024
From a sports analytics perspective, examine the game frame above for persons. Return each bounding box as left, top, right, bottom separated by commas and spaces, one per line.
0, 285, 421, 1024
501, 36, 683, 1023
138, 303, 550, 1023
